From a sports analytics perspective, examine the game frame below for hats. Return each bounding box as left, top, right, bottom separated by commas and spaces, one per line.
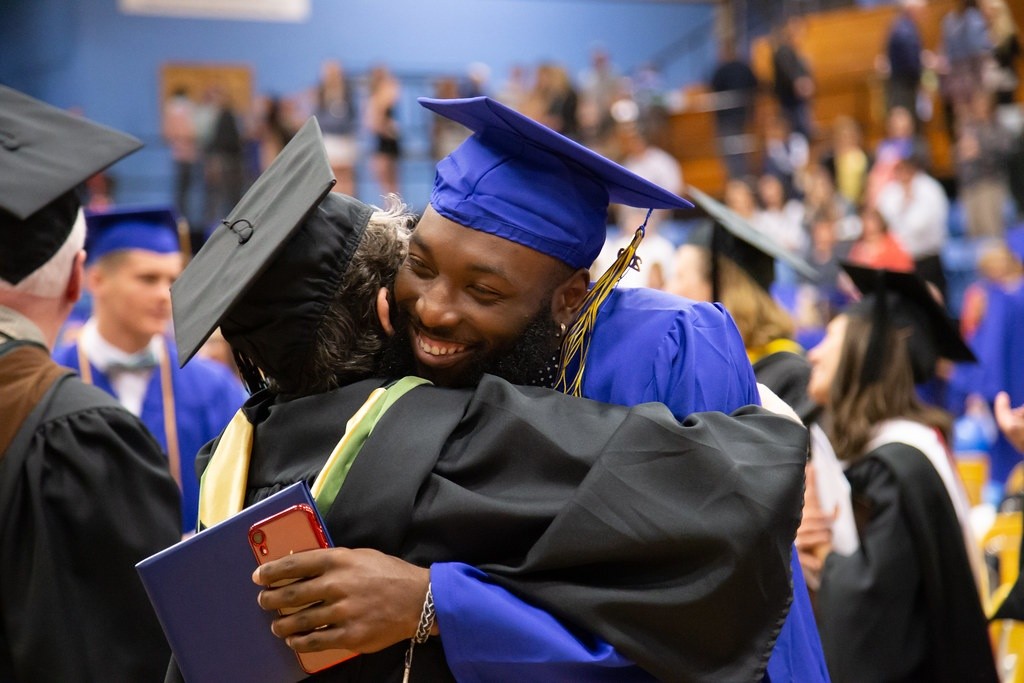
169, 115, 373, 370
420, 95, 693, 397
1, 83, 147, 287
684, 185, 821, 303
838, 259, 977, 365
80, 203, 181, 269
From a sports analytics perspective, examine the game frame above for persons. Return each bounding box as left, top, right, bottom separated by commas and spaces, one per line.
760, 262, 1004, 683
167, 115, 812, 682
3, 84, 184, 683
378, 94, 829, 682
57, 204, 251, 536
67, 0, 1022, 539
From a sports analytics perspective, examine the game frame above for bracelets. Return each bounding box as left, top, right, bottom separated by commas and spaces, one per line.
411, 575, 438, 646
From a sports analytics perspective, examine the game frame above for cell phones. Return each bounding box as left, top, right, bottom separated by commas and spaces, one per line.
248, 505, 363, 675
